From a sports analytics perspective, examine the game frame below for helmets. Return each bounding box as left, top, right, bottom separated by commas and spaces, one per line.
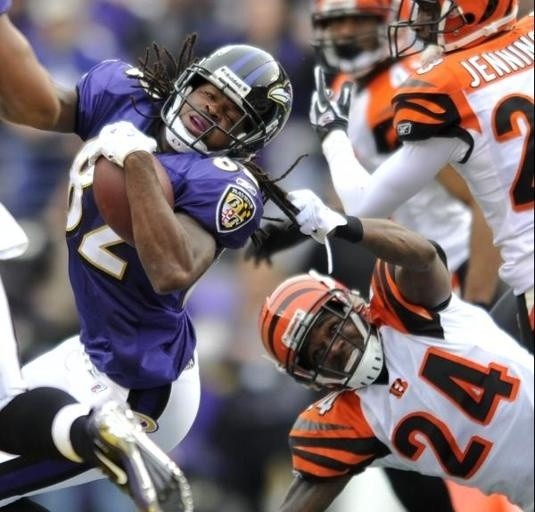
311, 0, 424, 73
257, 270, 383, 392
159, 43, 294, 160
386, 1, 518, 55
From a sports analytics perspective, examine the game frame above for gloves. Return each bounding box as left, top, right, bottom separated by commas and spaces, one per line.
308, 65, 354, 140
86, 120, 159, 167
287, 189, 363, 247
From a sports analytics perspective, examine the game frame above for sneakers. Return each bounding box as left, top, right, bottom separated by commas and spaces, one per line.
83, 399, 194, 511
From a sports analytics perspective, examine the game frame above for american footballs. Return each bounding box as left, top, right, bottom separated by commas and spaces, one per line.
93, 155, 174, 247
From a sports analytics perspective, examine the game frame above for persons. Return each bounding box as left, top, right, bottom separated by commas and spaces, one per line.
0, 0, 293, 512
311, 0, 473, 277
308, 0, 534, 365
0, 202, 194, 511
258, 189, 535, 512
